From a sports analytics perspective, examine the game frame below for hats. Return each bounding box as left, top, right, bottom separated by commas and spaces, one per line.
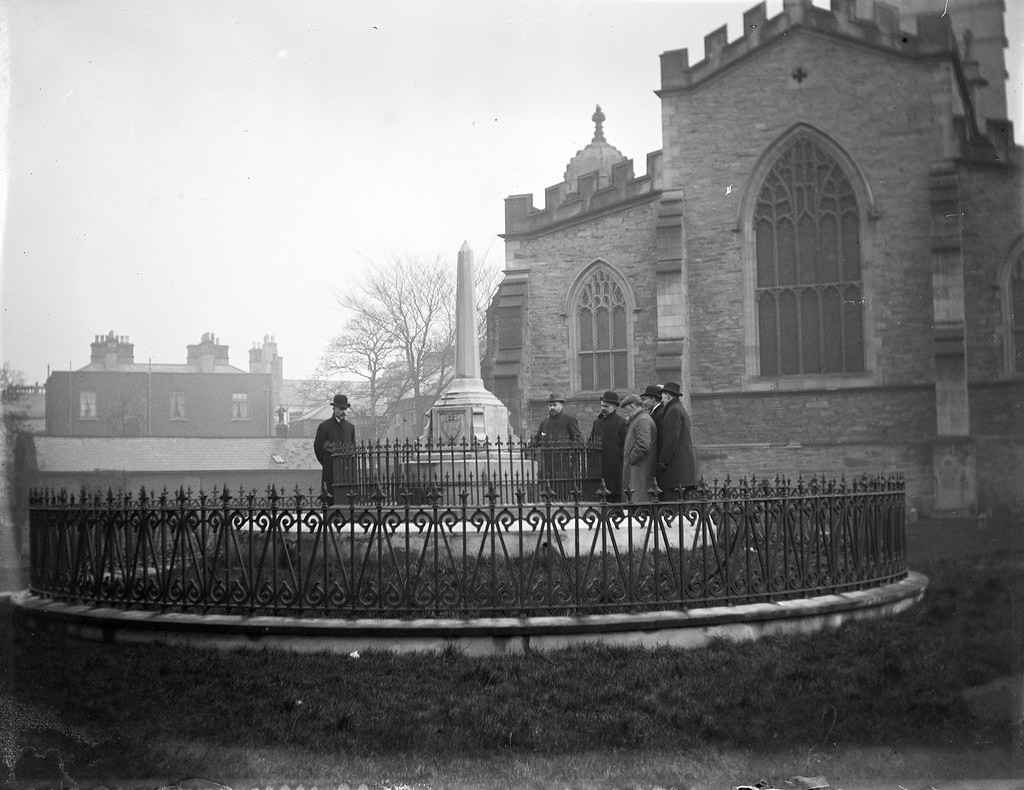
659, 382, 683, 396
330, 395, 350, 408
600, 391, 619, 406
640, 386, 662, 399
546, 394, 566, 403
620, 394, 642, 408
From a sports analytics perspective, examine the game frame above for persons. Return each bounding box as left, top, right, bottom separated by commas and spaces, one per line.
527, 394, 584, 502
275, 406, 289, 422
313, 394, 356, 506
587, 382, 698, 517
